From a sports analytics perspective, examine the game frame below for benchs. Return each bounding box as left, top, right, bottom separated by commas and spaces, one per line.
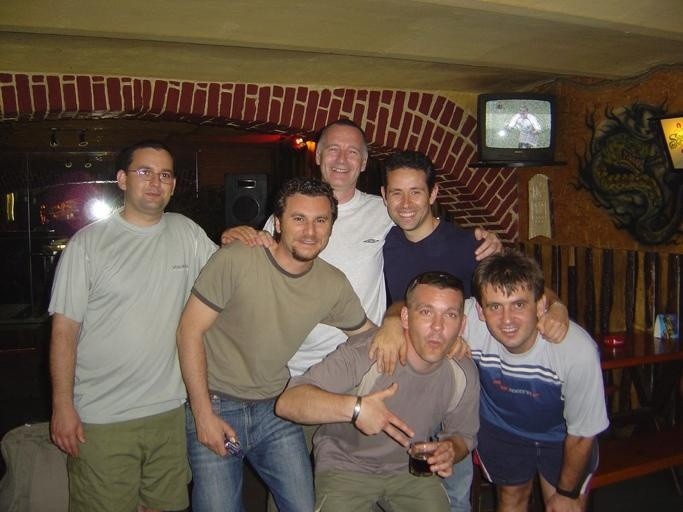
588, 421, 683, 491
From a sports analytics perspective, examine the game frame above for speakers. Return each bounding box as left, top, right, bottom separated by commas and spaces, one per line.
223, 172, 268, 227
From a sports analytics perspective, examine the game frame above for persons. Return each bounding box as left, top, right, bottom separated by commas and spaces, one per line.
44, 139, 264, 511
507, 104, 542, 148
175, 174, 476, 511
369, 247, 613, 510
380, 149, 569, 345
272, 269, 483, 511
258, 118, 503, 328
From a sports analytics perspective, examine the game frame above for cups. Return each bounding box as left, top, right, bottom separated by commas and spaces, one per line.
409, 440, 438, 478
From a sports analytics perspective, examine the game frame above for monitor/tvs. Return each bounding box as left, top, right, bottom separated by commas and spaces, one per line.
657, 112, 683, 173
477, 93, 557, 167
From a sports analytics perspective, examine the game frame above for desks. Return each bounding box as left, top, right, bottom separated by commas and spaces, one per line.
590, 330, 683, 497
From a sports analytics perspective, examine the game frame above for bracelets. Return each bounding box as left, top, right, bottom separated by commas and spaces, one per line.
556, 487, 580, 500
350, 395, 362, 424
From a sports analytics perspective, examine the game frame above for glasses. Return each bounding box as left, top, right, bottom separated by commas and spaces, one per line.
127, 167, 172, 184
408, 272, 463, 293
